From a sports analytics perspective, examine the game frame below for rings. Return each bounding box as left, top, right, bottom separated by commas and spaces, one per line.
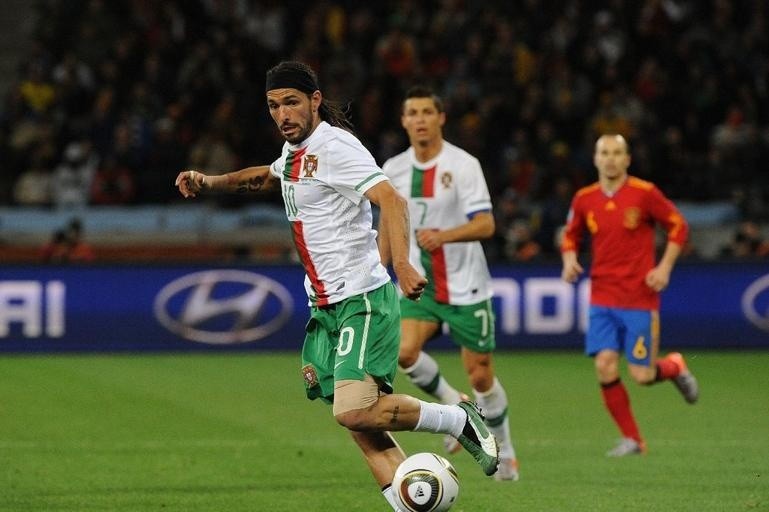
189, 171, 194, 180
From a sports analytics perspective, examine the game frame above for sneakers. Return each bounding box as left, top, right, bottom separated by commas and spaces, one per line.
667, 351, 698, 404
607, 438, 647, 457
494, 452, 519, 481
447, 392, 500, 476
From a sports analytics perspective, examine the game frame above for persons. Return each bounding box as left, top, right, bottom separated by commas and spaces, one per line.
1, 0, 769, 266
558, 130, 699, 460
367, 86, 524, 482
172, 61, 500, 512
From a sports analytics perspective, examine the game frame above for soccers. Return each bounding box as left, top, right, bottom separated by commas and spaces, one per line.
391, 452, 459, 512
